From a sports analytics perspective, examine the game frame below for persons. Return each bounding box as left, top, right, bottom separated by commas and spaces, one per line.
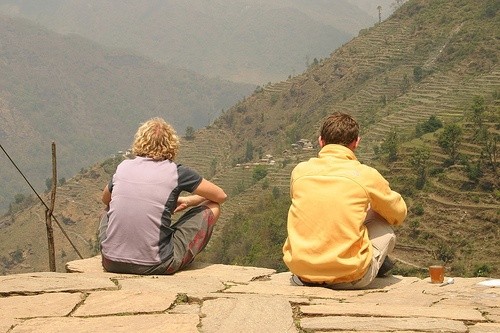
282, 112, 408, 289
97, 117, 230, 276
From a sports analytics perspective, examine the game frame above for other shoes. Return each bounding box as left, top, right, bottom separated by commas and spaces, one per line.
378, 256, 394, 276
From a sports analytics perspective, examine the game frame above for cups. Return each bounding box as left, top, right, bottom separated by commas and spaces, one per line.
430, 265, 445, 284
